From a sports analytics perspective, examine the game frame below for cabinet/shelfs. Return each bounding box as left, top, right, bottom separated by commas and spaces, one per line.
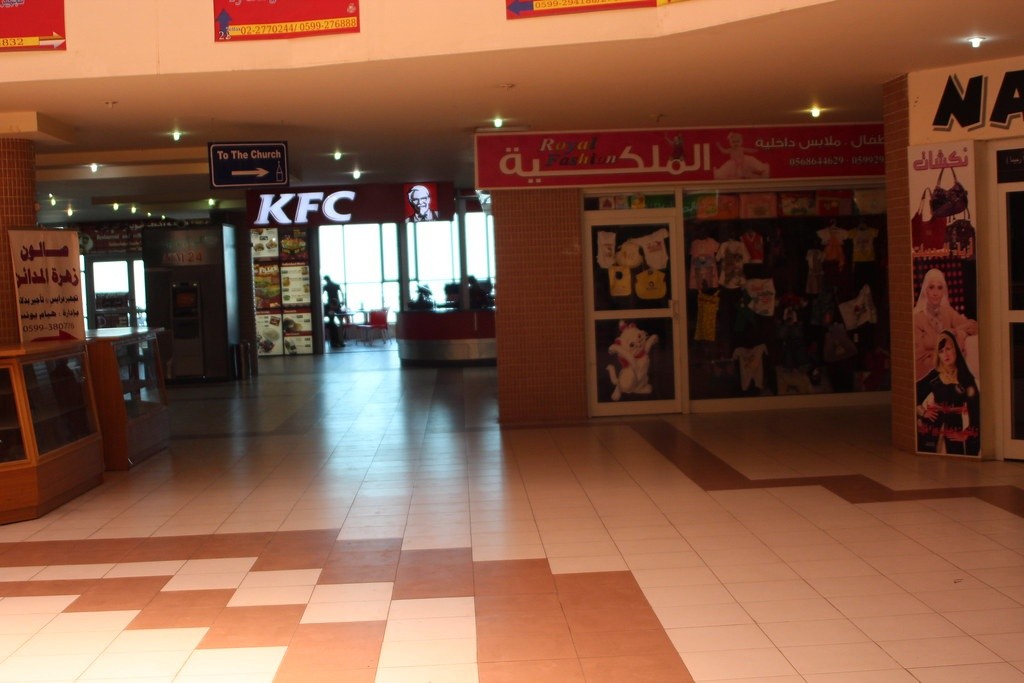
83, 327, 170, 470
0, 340, 106, 526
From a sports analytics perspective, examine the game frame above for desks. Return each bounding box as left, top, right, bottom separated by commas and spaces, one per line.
354, 309, 372, 343
333, 312, 354, 345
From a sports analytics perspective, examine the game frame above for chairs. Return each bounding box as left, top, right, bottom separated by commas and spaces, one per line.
325, 307, 392, 346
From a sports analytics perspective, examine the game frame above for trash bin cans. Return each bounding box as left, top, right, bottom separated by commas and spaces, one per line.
234, 339, 256, 382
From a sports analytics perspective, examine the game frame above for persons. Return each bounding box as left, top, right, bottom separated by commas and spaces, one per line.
915, 330, 979, 456
913, 268, 978, 380
322, 276, 344, 313
